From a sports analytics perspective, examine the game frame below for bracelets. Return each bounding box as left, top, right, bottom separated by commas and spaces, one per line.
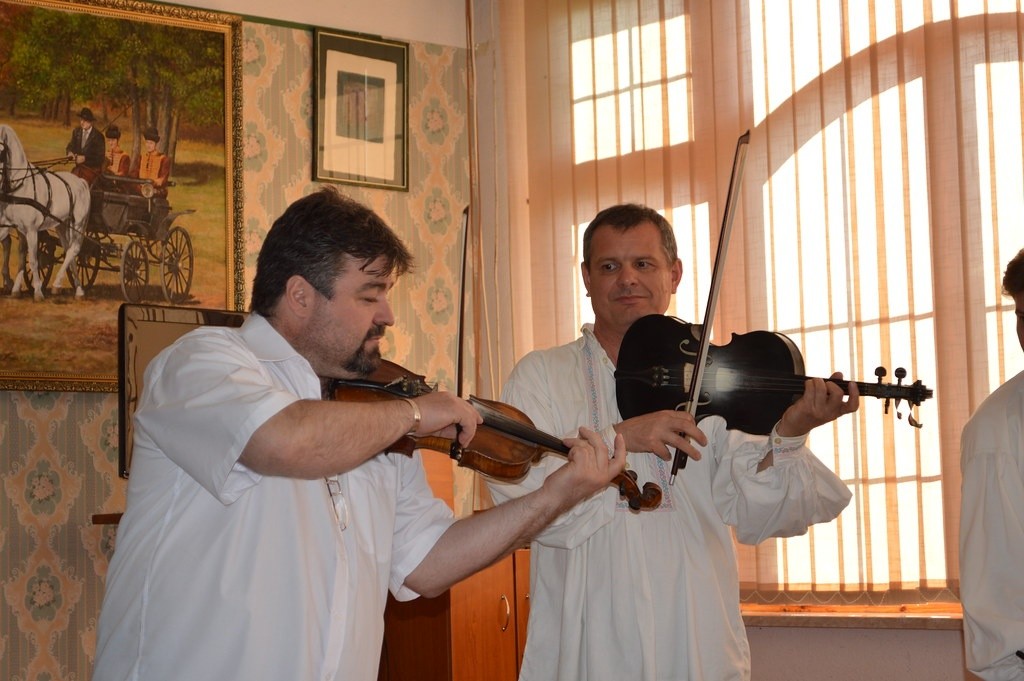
400, 397, 420, 437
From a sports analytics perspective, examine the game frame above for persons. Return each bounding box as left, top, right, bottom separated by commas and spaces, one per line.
958, 246, 1024, 680
482, 204, 858, 680
92, 189, 627, 680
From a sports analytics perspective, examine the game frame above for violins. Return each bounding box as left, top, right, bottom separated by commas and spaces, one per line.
333, 360, 662, 514
612, 313, 934, 437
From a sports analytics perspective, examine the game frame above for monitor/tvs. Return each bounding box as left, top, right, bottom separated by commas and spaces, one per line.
118, 302, 251, 480
